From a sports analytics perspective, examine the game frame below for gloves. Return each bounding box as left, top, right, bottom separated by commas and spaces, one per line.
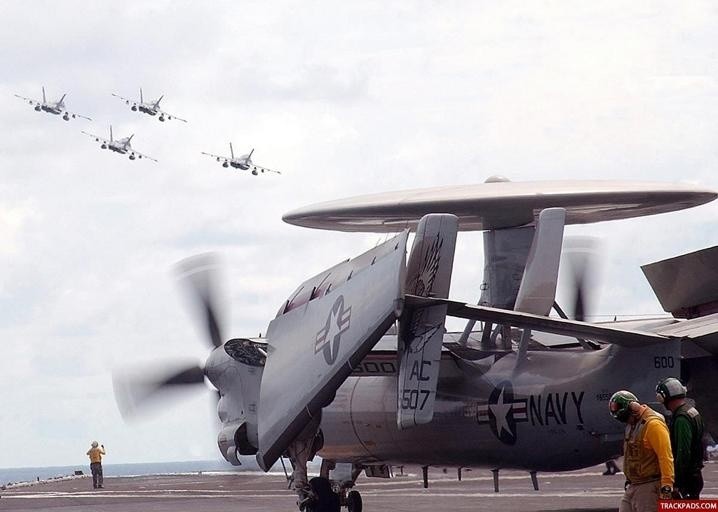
660, 491, 671, 500
625, 481, 629, 489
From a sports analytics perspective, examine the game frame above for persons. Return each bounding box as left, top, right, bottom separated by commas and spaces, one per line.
87, 441, 106, 489
653, 377, 707, 501
609, 390, 676, 511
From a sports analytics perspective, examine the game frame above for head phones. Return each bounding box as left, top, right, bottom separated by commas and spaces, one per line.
655, 376, 688, 402
628, 401, 641, 416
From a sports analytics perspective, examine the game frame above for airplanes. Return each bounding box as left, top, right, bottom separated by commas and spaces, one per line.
105, 175, 718, 512
19, 80, 286, 181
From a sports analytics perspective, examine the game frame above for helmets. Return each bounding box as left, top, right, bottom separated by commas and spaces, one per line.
92, 442, 97, 448
656, 378, 686, 409
609, 391, 638, 424
